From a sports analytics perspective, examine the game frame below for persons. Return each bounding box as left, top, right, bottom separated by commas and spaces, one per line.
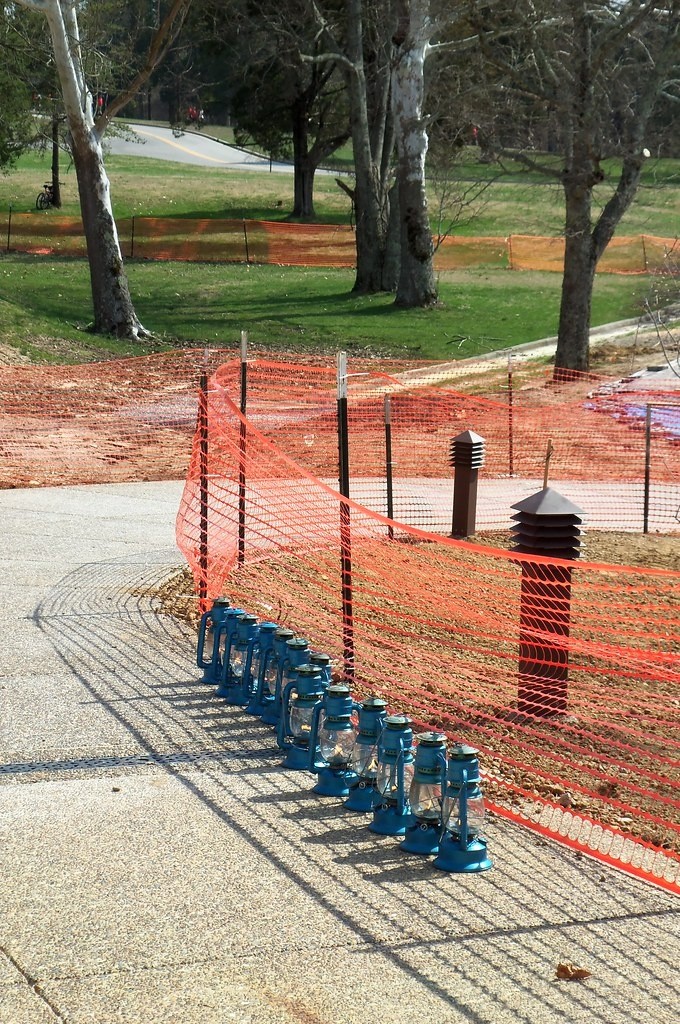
97, 95, 103, 116
189, 106, 204, 127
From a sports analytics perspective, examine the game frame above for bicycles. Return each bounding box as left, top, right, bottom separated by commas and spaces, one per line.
35, 182, 66, 210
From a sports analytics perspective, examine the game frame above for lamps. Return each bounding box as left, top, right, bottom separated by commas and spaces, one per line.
447, 430, 487, 469
508, 487, 589, 570
196, 596, 493, 873
